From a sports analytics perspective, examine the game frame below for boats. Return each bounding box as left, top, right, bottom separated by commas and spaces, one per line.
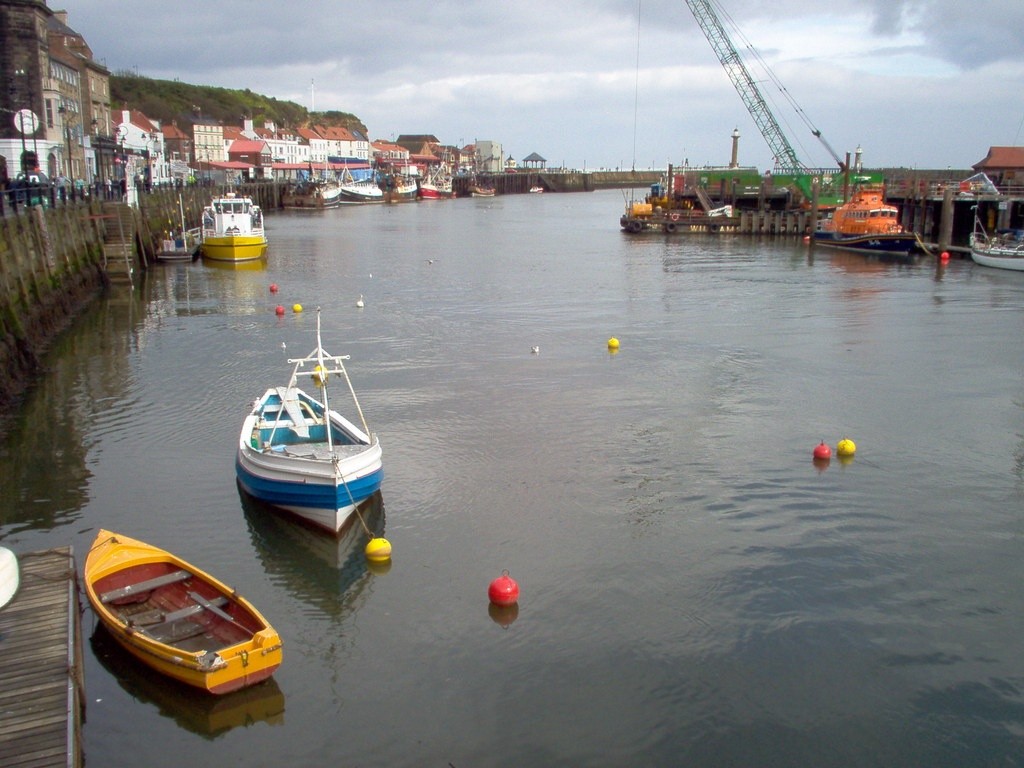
281, 177, 342, 209
88, 618, 287, 742
968, 232, 1024, 272
467, 186, 496, 197
236, 476, 385, 624
200, 192, 268, 261
419, 161, 453, 199
85, 529, 283, 696
530, 186, 544, 193
339, 153, 418, 204
156, 232, 200, 263
814, 188, 918, 259
235, 305, 384, 542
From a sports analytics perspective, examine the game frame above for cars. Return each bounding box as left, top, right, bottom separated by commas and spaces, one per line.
507, 169, 517, 173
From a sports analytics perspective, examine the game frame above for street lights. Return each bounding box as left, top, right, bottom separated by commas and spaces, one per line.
58, 104, 76, 203
114, 126, 128, 183
14, 65, 39, 171
141, 133, 152, 194
120, 135, 126, 178
90, 117, 106, 199
